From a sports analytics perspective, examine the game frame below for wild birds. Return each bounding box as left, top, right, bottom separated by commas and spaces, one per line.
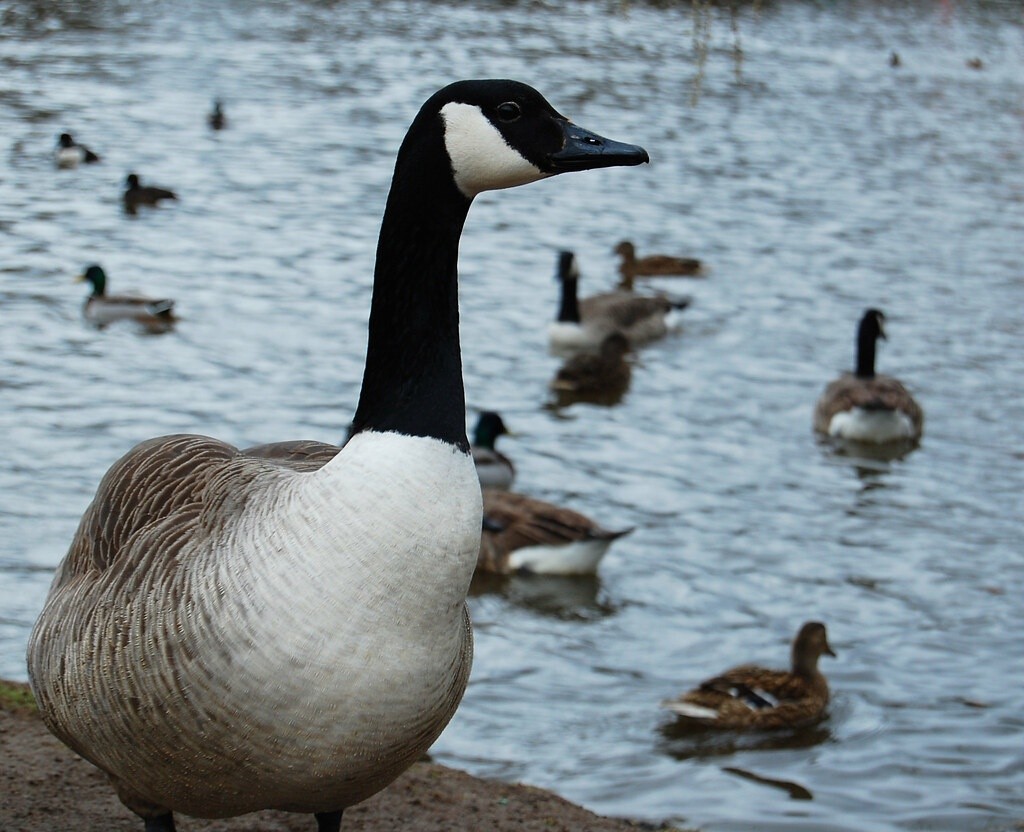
27, 51, 990, 832
